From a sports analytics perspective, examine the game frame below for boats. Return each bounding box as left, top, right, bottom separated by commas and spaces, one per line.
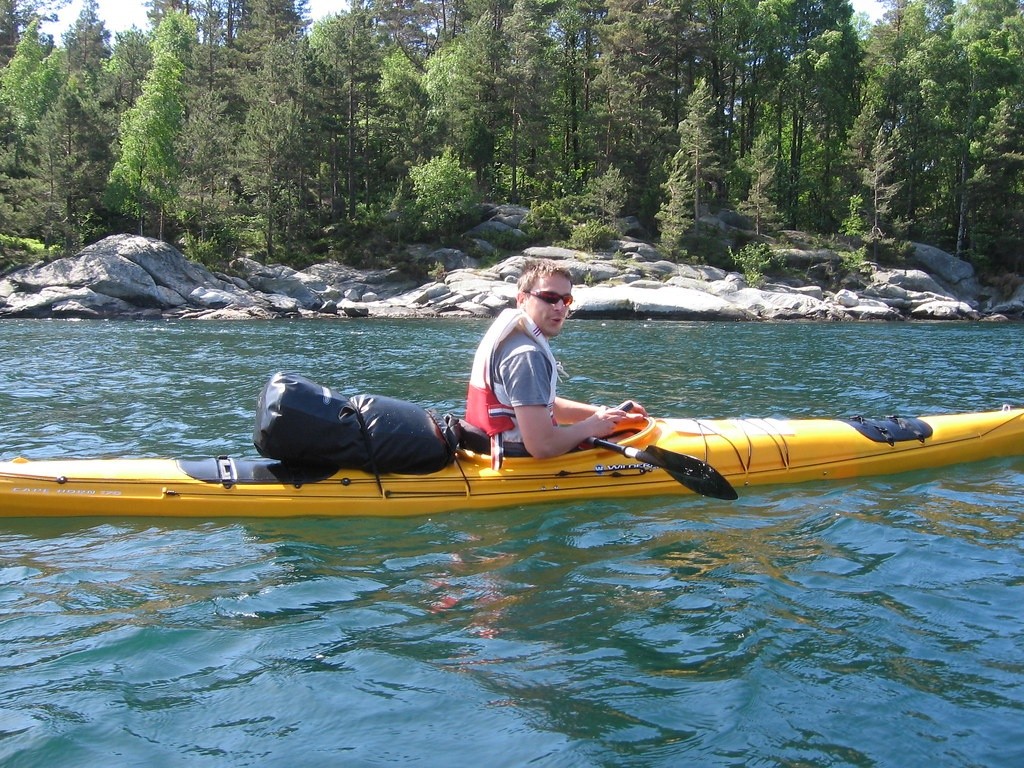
0, 370, 1024, 517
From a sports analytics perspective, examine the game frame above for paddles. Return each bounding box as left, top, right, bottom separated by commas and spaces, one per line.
586, 436, 739, 501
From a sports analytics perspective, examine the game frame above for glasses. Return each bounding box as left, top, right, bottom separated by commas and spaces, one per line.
524, 289, 573, 306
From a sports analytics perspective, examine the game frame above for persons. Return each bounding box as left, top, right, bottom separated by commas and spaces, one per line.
466, 261, 648, 460
